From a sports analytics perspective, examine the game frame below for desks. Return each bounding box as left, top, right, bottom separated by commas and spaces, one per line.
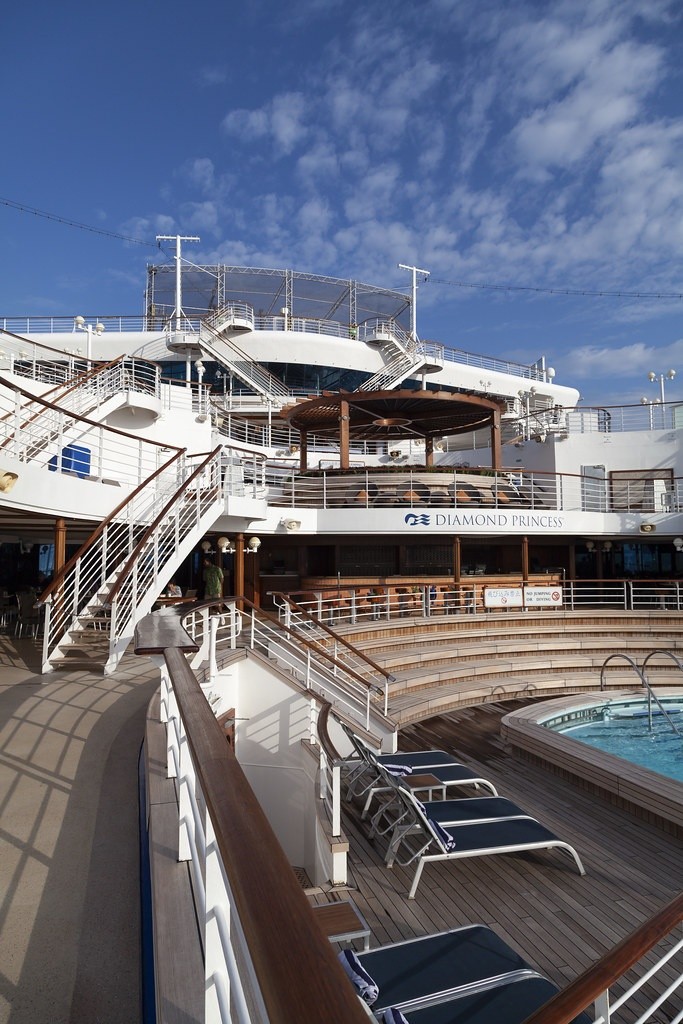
155, 597, 197, 610
398, 774, 446, 824
312, 901, 371, 952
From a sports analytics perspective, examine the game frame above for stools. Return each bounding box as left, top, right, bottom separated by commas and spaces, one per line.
303, 584, 558, 630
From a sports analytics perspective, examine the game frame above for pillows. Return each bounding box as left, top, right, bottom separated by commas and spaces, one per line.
498, 492, 510, 502
457, 489, 470, 502
357, 489, 371, 501
404, 491, 421, 501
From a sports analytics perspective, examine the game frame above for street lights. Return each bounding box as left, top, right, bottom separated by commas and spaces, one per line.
641, 397, 660, 429
76, 314, 105, 393
518, 386, 537, 442
649, 369, 675, 430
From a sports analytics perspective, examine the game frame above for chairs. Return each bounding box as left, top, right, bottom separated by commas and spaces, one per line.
356, 974, 577, 1024
397, 481, 430, 508
2, 587, 46, 639
447, 480, 495, 509
491, 481, 531, 509
332, 711, 587, 900
345, 481, 377, 509
186, 588, 196, 598
517, 485, 550, 509
359, 923, 543, 1014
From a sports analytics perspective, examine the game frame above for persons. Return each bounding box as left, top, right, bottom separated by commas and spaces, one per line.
203, 558, 225, 627
167, 582, 182, 597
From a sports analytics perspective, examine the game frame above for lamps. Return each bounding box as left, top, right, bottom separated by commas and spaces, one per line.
243, 536, 260, 552
203, 541, 216, 554
601, 541, 612, 552
673, 538, 683, 551
586, 542, 597, 552
218, 537, 237, 553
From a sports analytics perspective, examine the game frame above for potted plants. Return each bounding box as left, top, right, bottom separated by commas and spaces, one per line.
411, 585, 422, 598
367, 590, 379, 601
